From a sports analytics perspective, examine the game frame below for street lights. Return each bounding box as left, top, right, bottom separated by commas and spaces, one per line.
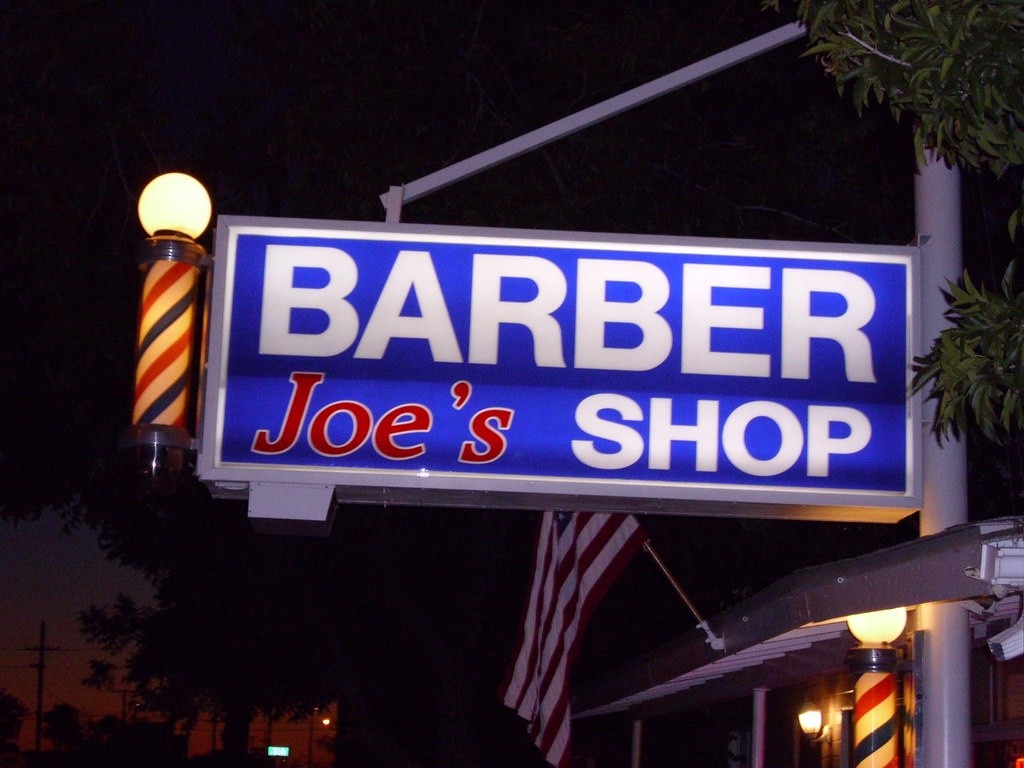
108, 687, 137, 724
309, 708, 319, 766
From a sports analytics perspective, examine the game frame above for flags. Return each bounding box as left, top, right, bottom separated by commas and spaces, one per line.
498, 509, 649, 768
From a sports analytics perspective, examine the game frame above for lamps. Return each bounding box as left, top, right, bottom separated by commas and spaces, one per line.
797, 693, 832, 749
